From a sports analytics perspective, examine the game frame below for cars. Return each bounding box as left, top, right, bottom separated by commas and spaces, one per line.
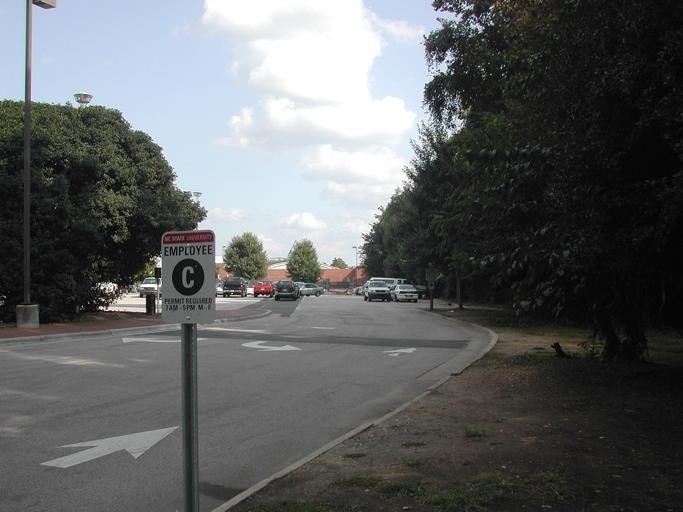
254, 280, 325, 301
139, 277, 162, 298
353, 277, 428, 303
216, 276, 248, 298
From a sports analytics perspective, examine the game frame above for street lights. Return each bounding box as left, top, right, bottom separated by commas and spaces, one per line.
352, 245, 361, 287
16, 0, 57, 329
